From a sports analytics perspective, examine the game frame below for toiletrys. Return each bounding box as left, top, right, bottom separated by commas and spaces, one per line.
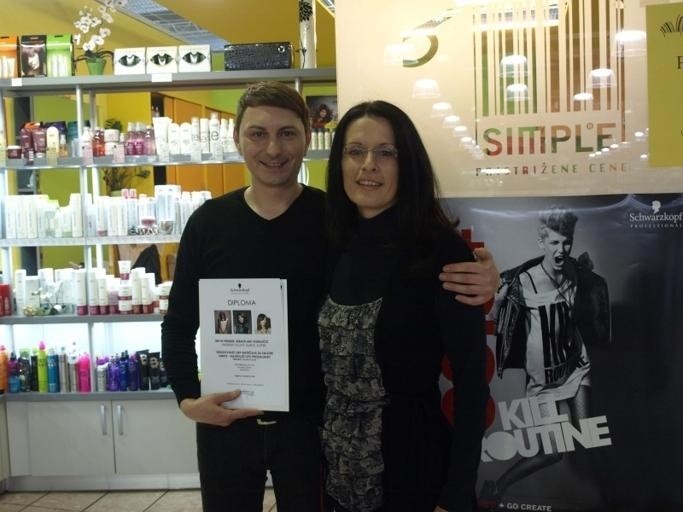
0, 114, 337, 393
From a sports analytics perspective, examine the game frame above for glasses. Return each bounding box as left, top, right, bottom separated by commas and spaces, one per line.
342, 143, 399, 160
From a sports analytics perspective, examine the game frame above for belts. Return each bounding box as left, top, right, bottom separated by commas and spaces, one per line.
247, 417, 277, 426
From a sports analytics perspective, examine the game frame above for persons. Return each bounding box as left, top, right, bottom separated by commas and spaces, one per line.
317, 101, 490, 512
162, 82, 501, 511
479, 209, 611, 501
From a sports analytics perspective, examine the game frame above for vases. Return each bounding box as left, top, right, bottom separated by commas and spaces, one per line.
85, 58, 106, 75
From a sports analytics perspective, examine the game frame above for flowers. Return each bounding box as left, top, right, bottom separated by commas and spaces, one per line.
73, 0, 128, 68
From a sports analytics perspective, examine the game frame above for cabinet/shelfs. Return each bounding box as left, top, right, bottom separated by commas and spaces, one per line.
0, 67, 339, 493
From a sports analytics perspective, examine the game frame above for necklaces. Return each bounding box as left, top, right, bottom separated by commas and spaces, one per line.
542, 262, 563, 287
